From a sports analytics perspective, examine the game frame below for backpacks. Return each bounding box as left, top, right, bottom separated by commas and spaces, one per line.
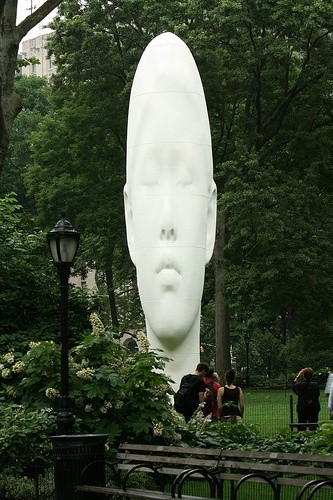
174, 375, 201, 414
201, 379, 218, 413
298, 382, 317, 417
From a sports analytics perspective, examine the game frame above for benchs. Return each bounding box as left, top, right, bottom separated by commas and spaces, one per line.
77, 443, 333, 500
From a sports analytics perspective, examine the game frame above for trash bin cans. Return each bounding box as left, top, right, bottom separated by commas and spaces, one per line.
48, 433, 109, 500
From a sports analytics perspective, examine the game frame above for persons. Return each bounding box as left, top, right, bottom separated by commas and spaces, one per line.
179, 362, 222, 423
123, 32, 218, 407
216, 369, 245, 423
292, 367, 321, 432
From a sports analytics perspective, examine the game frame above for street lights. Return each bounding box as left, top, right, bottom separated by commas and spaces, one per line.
46, 212, 82, 433
